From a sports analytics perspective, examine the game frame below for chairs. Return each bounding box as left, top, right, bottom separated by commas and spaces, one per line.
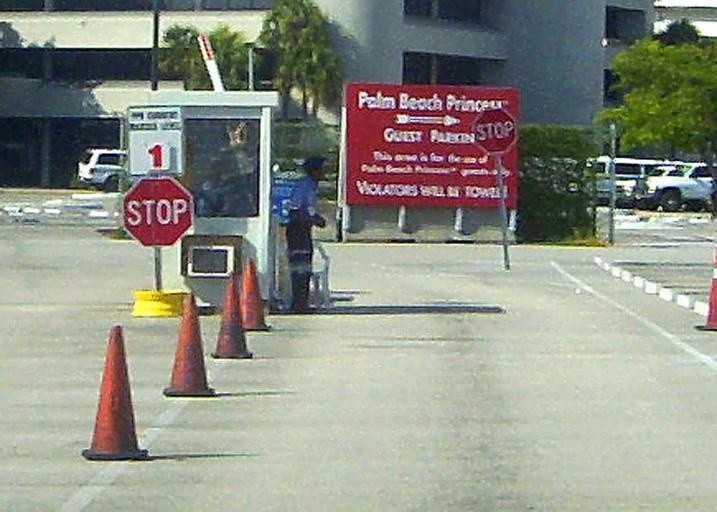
281, 243, 329, 311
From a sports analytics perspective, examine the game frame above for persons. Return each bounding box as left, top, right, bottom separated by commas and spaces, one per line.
282, 156, 328, 314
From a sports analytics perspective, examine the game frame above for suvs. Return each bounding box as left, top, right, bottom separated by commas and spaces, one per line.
76, 145, 130, 194
528, 154, 717, 212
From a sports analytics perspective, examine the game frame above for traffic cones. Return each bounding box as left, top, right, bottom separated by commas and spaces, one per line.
76, 319, 153, 460
159, 292, 220, 398
235, 258, 278, 335
692, 247, 717, 333
205, 269, 258, 363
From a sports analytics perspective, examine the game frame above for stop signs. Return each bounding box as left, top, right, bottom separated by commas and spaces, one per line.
470, 107, 517, 155
119, 176, 191, 248
